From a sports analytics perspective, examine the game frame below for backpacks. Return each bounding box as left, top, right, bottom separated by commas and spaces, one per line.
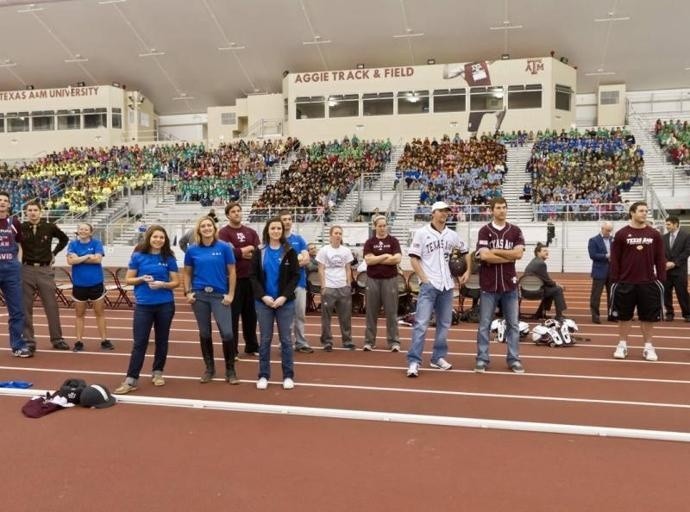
460, 305, 496, 323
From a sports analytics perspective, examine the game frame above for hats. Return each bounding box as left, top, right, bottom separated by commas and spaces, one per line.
80, 383, 115, 408
431, 201, 452, 214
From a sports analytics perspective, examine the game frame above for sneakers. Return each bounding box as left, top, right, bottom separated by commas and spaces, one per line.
152, 372, 165, 386
430, 358, 452, 370
295, 347, 313, 353
101, 339, 115, 350
364, 343, 376, 351
474, 359, 486, 373
614, 345, 628, 359
325, 344, 333, 352
643, 347, 658, 361
247, 347, 259, 356
12, 347, 34, 358
200, 369, 215, 382
283, 378, 295, 390
407, 362, 419, 378
112, 378, 138, 394
391, 345, 401, 352
256, 377, 269, 390
344, 344, 356, 348
73, 341, 84, 352
508, 361, 525, 373
24, 346, 36, 352
224, 373, 240, 385
53, 341, 70, 350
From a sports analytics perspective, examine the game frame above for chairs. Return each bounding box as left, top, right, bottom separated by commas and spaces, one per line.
408, 272, 421, 311
518, 273, 548, 321
308, 271, 322, 312
356, 271, 367, 310
398, 272, 407, 319
50, 266, 76, 306
116, 268, 135, 310
102, 268, 132, 310
456, 273, 482, 321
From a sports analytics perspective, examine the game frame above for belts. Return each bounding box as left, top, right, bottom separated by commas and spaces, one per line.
23, 262, 49, 267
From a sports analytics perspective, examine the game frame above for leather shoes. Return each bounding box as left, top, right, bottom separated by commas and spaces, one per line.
665, 315, 674, 321
592, 320, 601, 324
608, 316, 618, 321
684, 316, 689, 322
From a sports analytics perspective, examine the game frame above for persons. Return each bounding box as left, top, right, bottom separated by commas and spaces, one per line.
1, 136, 302, 222
115, 205, 568, 397
296, 135, 392, 222
588, 201, 690, 360
393, 118, 690, 223
66, 223, 115, 352
14, 202, 71, 352
1, 191, 34, 358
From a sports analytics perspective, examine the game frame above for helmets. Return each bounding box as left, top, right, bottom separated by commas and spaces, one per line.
544, 319, 560, 329
491, 319, 506, 342
558, 319, 579, 345
518, 321, 529, 337
449, 257, 467, 277
531, 325, 563, 346
